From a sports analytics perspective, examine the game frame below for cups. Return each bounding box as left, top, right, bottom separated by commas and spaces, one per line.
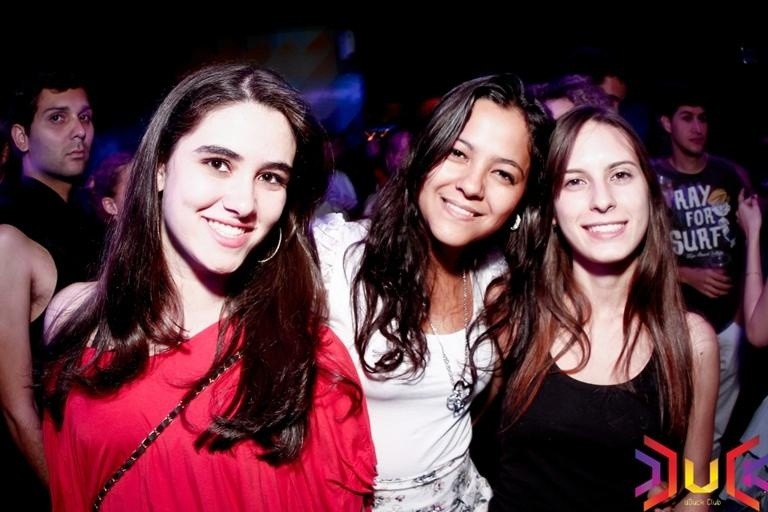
712, 256, 730, 276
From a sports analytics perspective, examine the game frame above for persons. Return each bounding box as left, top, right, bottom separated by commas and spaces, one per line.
1, 66, 768, 511
